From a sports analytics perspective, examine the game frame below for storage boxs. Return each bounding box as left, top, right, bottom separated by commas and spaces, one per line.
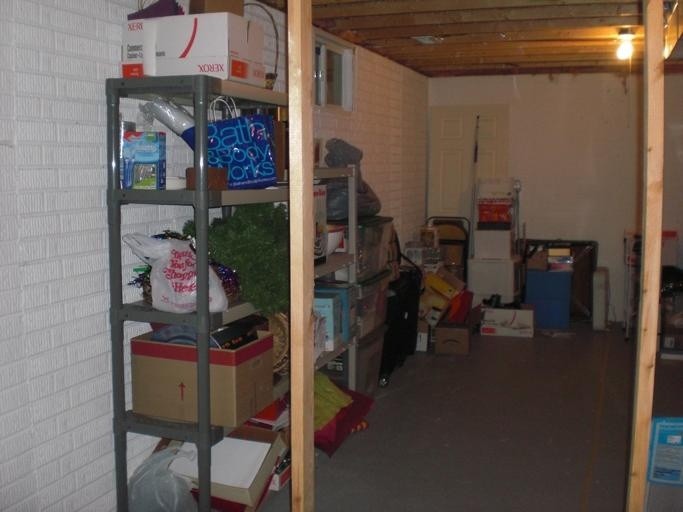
415, 183, 574, 355
151, 428, 287, 507
130, 325, 274, 430
320, 215, 393, 400
122, 0, 266, 88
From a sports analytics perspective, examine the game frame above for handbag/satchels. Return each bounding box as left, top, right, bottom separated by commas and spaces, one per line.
179, 112, 278, 190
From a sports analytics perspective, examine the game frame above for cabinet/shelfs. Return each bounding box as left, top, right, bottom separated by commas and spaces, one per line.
104, 74, 290, 512
